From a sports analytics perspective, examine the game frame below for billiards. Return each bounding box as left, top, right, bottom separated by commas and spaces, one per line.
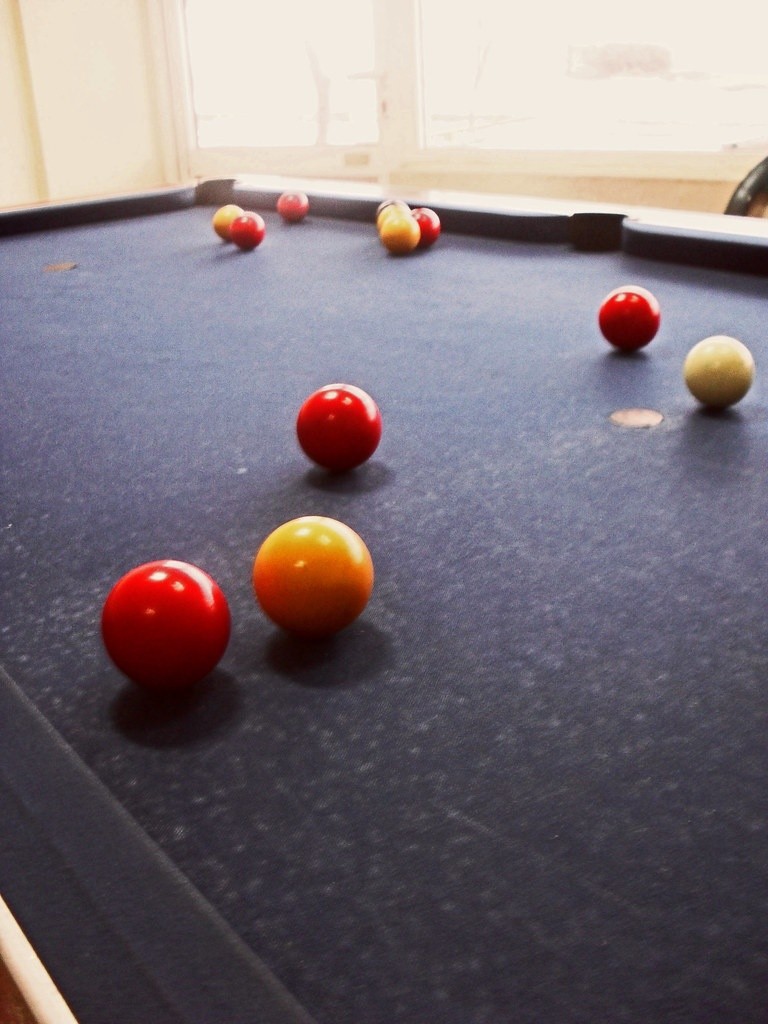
295, 382, 383, 475
375, 199, 442, 254
99, 559, 231, 689
277, 191, 308, 223
212, 203, 266, 251
248, 514, 376, 640
598, 285, 662, 352
681, 335, 757, 410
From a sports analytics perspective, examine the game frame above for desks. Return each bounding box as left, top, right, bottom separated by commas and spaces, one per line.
0, 169, 768, 1024
